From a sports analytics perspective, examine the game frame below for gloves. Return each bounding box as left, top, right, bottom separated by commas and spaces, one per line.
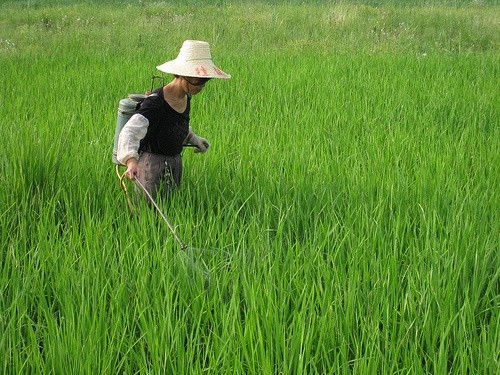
188, 134, 209, 153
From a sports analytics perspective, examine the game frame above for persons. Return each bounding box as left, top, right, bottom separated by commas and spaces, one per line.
117, 39, 232, 210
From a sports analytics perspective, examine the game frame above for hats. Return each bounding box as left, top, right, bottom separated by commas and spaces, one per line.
156, 39, 231, 79
